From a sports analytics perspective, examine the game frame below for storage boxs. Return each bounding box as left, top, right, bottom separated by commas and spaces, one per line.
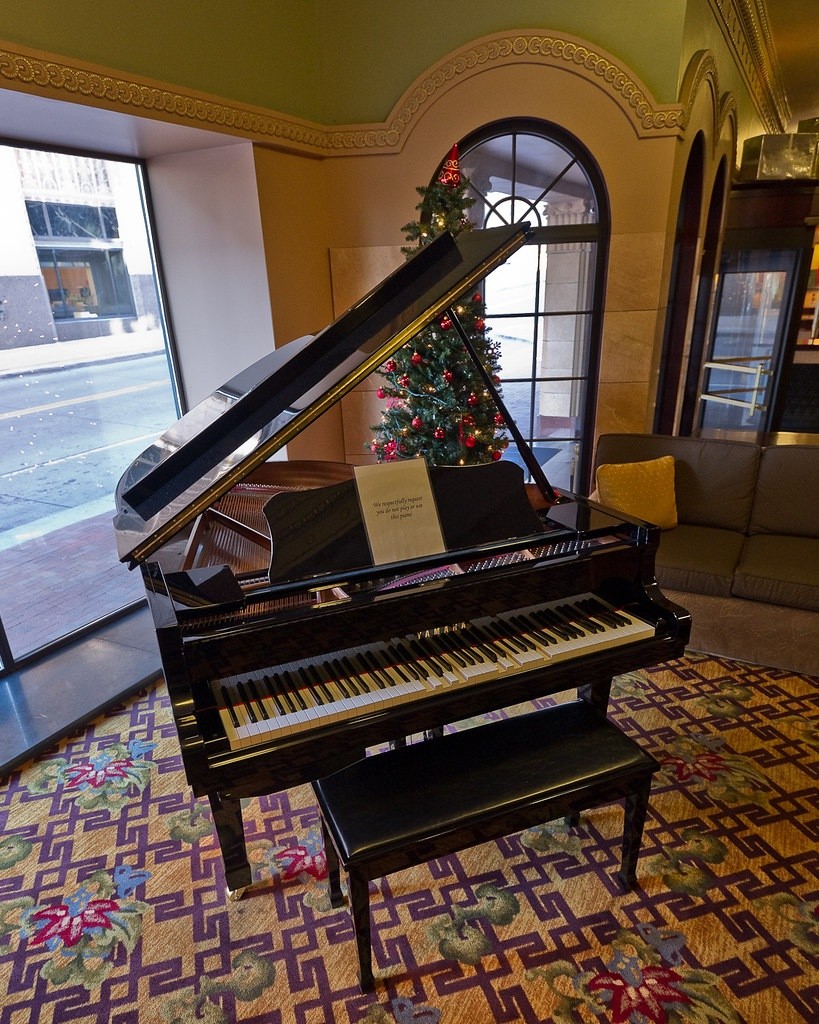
740, 133, 818, 182
798, 116, 819, 133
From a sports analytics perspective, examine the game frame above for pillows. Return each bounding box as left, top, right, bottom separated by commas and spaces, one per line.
595, 456, 678, 531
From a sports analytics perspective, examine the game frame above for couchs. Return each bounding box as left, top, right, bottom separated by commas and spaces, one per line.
586, 432, 819, 677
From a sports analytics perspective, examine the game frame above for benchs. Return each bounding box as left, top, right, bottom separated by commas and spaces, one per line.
309, 697, 663, 996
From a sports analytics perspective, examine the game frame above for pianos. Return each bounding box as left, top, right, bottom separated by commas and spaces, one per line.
109, 222, 694, 891
